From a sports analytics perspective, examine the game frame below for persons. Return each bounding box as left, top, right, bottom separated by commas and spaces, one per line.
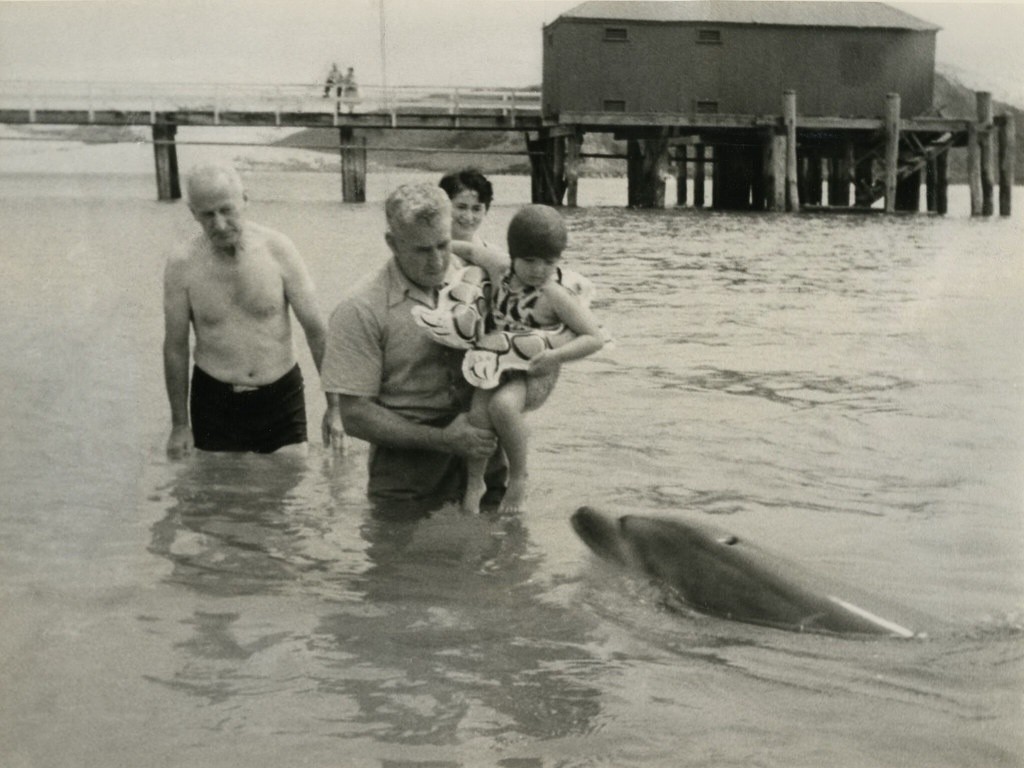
452, 204, 604, 520
323, 64, 360, 114
320, 183, 559, 520
440, 166, 489, 265
161, 161, 350, 465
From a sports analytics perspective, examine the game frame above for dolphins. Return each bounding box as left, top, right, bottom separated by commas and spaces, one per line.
569, 506, 959, 638
